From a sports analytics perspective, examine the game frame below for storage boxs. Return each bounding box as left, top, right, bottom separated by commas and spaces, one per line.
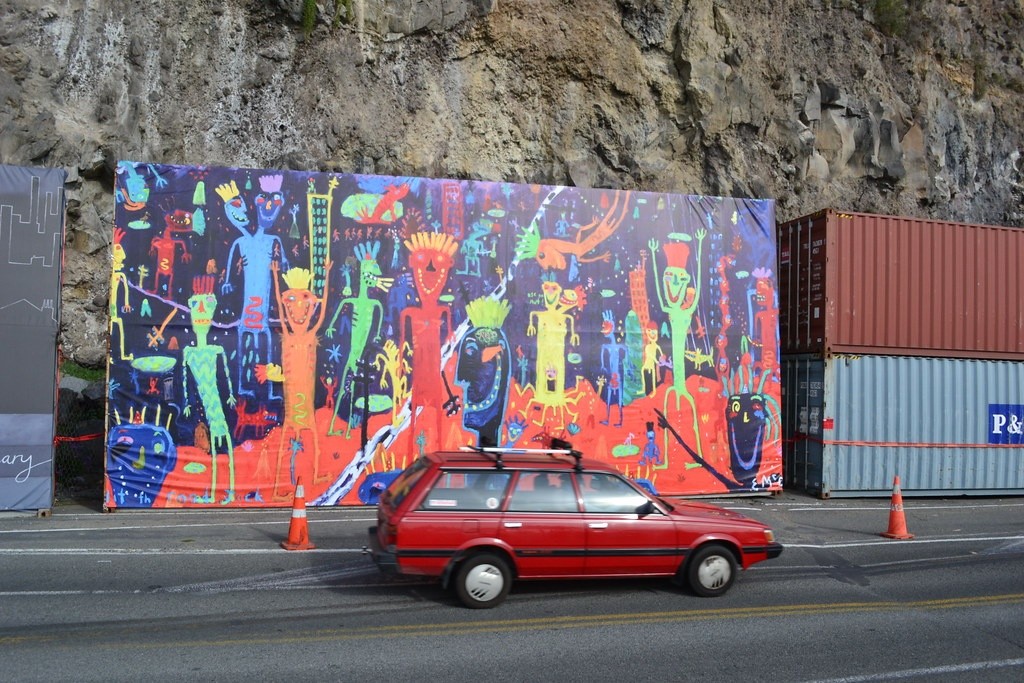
776, 209, 1024, 359
781, 353, 1024, 501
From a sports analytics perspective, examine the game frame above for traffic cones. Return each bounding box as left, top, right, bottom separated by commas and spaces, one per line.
278, 476, 316, 550
881, 475, 915, 541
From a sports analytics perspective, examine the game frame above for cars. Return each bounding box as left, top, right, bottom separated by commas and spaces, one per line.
367, 441, 784, 609
